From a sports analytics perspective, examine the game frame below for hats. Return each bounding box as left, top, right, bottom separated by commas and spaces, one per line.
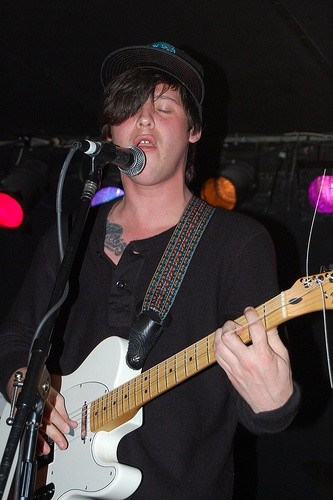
100, 41, 204, 122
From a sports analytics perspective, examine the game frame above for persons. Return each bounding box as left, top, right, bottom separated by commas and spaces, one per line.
0, 42, 302, 500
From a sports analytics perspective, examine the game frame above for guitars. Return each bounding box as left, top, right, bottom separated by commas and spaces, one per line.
1, 260, 333, 500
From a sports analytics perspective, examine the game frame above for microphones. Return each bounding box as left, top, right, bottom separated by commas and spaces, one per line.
72, 141, 146, 177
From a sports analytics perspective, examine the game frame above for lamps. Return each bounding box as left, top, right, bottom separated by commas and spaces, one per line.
0, 160, 53, 229
299, 167, 333, 219
199, 156, 259, 212
